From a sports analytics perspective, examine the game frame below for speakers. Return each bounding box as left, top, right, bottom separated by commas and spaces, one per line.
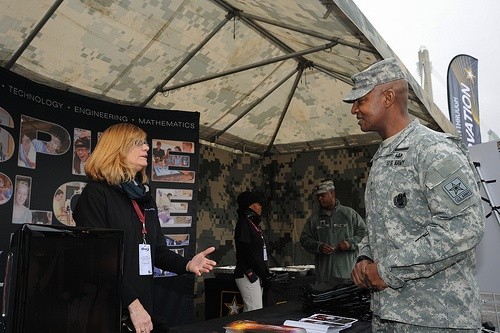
6, 223, 124, 333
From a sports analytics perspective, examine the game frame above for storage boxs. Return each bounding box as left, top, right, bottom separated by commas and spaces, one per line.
7, 222, 126, 333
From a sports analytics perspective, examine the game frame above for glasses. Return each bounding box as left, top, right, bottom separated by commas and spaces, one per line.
130, 140, 147, 146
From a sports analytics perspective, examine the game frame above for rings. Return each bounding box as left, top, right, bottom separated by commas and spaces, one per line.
142, 327, 146, 332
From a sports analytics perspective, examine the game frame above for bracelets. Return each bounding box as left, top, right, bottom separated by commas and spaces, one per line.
356, 255, 374, 263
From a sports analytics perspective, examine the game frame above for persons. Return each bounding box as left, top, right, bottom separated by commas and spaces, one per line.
232, 189, 272, 313
342, 58, 486, 333
300, 180, 367, 279
148, 135, 194, 226
73, 123, 216, 333
0, 108, 90, 225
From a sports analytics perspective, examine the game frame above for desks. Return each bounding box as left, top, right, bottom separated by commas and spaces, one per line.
204, 272, 317, 306
169, 300, 372, 333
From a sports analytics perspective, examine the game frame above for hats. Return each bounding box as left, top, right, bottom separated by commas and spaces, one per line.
237, 191, 258, 207
342, 56, 406, 104
314, 180, 334, 196
